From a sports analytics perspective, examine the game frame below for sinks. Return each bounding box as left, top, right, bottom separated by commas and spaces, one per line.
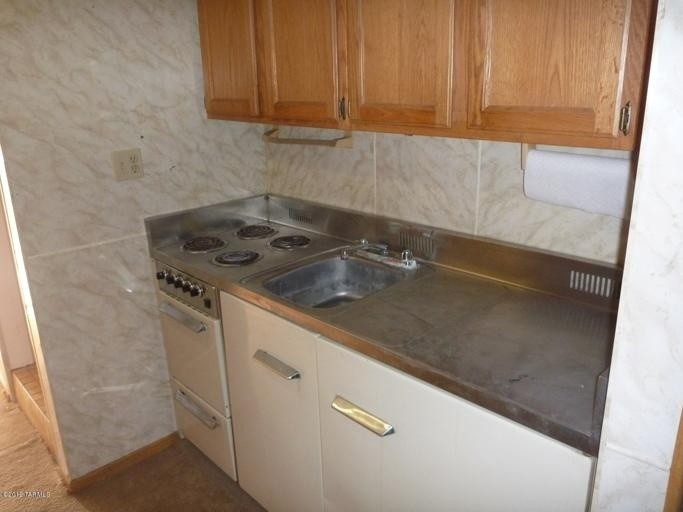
238, 243, 435, 316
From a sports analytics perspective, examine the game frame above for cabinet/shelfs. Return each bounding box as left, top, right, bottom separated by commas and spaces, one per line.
153, 286, 596, 512
266, 1, 658, 152
196, 0, 266, 125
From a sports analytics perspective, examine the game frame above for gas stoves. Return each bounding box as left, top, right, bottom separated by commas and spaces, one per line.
156, 220, 355, 282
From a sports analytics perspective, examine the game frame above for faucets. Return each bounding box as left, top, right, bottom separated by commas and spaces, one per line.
340, 242, 390, 261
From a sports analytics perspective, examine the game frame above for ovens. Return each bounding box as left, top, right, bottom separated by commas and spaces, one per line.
152, 259, 237, 482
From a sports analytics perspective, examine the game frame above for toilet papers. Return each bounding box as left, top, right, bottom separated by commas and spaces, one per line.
522, 149, 639, 222
